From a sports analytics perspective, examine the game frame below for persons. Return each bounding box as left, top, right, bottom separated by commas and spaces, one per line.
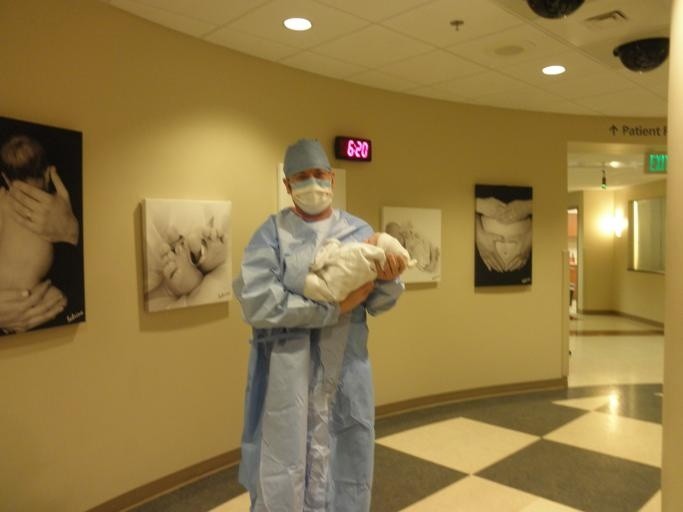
1, 134, 84, 333
386, 221, 439, 272
158, 225, 228, 296
232, 137, 404, 511
475, 185, 532, 284
304, 231, 417, 304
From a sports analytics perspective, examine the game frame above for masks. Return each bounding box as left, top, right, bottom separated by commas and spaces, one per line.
285, 178, 334, 216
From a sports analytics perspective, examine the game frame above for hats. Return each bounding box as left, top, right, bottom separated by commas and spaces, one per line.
284, 139, 331, 180
